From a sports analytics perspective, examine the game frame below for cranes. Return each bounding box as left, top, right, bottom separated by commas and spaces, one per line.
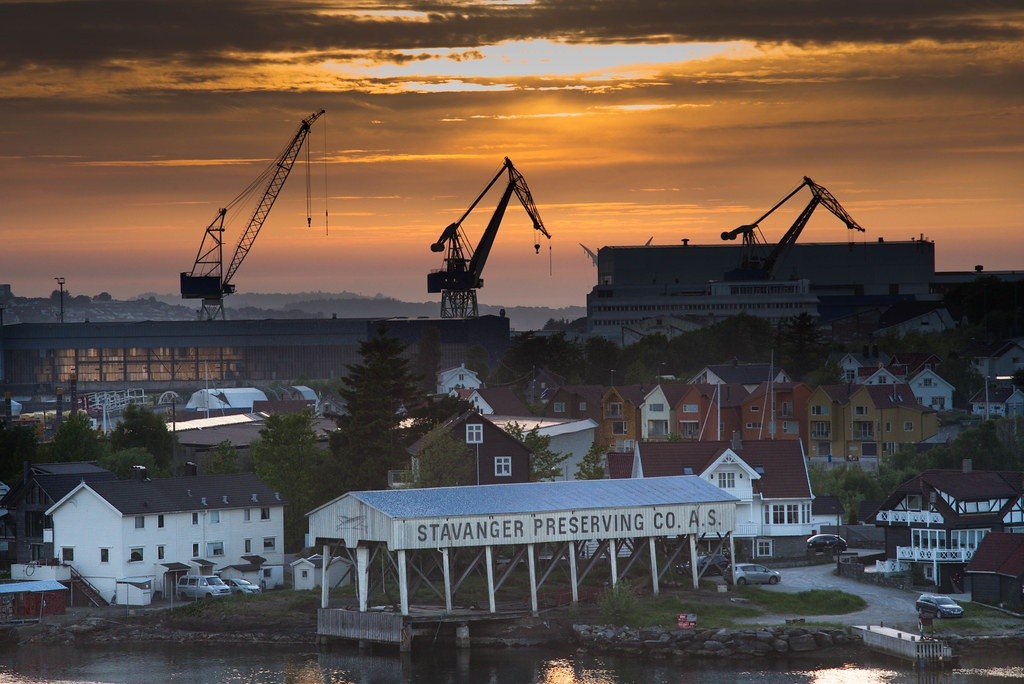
427, 156, 552, 318
719, 175, 866, 281
180, 107, 329, 320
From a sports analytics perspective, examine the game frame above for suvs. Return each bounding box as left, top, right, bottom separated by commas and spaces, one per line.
915, 594, 964, 619
806, 533, 848, 555
724, 563, 782, 585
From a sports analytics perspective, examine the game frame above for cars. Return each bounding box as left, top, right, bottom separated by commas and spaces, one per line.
222, 577, 261, 596
675, 554, 729, 576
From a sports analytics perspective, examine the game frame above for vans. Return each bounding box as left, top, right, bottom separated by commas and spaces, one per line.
177, 575, 232, 601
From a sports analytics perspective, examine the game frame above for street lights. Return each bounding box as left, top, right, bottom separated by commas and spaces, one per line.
657, 362, 667, 385
54, 277, 65, 322
610, 369, 617, 387
985, 375, 992, 422
